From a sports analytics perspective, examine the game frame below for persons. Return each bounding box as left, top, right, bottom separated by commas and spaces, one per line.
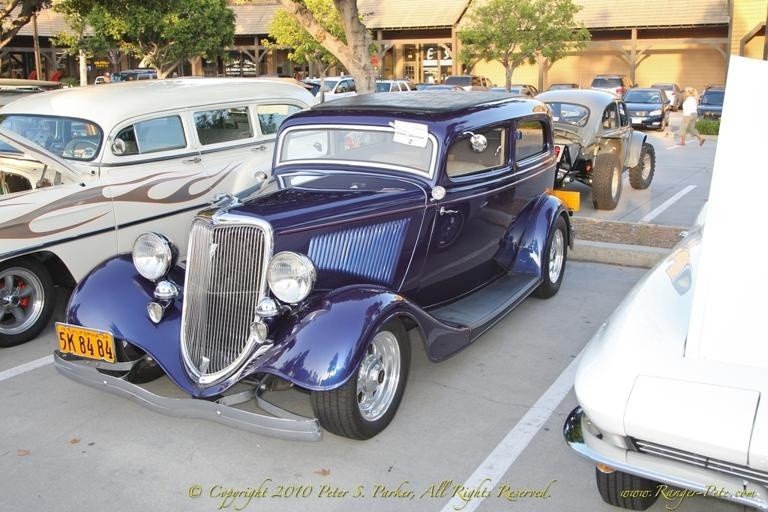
674, 87, 706, 147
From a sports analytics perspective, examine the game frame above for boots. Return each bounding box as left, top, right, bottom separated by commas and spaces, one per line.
698, 136, 705, 145
676, 136, 685, 146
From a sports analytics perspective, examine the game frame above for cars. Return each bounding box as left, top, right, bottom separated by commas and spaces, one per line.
563, 53, 768, 511
0, 59, 725, 443
